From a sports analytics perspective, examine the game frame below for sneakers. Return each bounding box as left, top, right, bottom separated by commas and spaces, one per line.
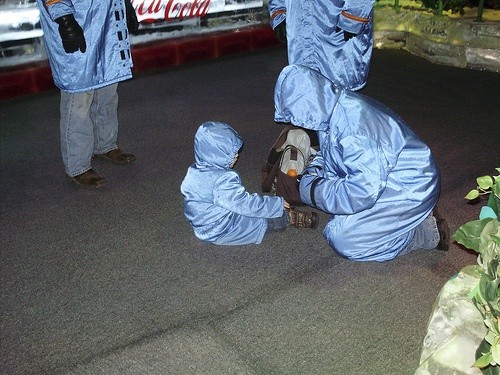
289, 207, 319, 229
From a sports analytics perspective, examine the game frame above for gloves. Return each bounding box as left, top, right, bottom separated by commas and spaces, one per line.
55, 13, 87, 54
125, 0, 138, 35
276, 20, 287, 46
336, 26, 358, 41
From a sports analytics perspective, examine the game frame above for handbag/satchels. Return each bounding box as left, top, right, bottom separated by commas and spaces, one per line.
262, 140, 306, 206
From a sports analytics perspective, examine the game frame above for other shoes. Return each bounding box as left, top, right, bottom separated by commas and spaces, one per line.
436, 219, 452, 250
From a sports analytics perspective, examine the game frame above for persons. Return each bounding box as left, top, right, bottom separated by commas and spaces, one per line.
38, 0, 139, 190
179, 120, 320, 246
274, 63, 450, 260
267, 0, 375, 93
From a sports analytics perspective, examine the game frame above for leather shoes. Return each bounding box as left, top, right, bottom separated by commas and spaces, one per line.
72, 169, 107, 188
94, 147, 136, 164
